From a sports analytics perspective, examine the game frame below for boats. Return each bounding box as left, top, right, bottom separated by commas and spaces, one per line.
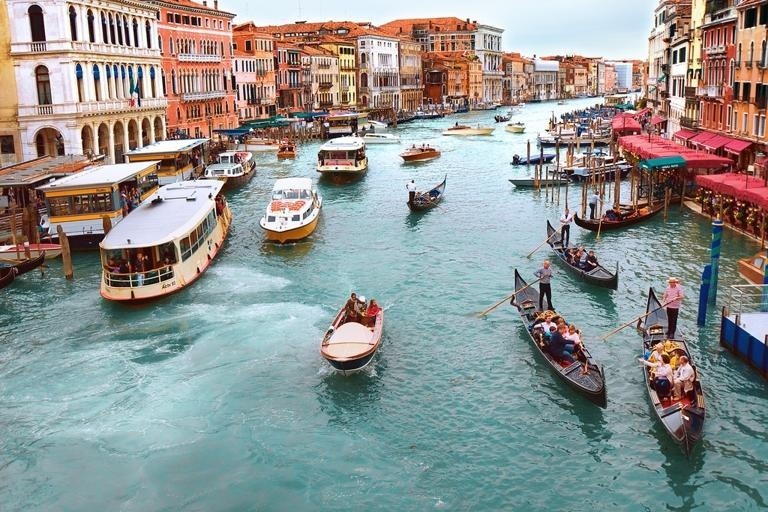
732, 247, 767, 294
570, 201, 666, 231
681, 173, 768, 248
211, 109, 388, 151
383, 100, 503, 125
315, 134, 369, 185
316, 290, 385, 375
360, 133, 401, 145
277, 144, 296, 160
510, 154, 557, 166
35, 159, 162, 251
494, 113, 513, 123
0, 249, 46, 278
557, 99, 570, 105
544, 218, 619, 292
406, 174, 448, 213
259, 177, 323, 244
204, 149, 257, 188
637, 285, 707, 462
505, 121, 526, 134
537, 93, 734, 204
442, 121, 496, 136
511, 265, 608, 412
505, 107, 522, 115
399, 147, 441, 164
0, 243, 63, 260
97, 178, 233, 305
518, 102, 526, 108
508, 174, 569, 186
121, 138, 210, 188
0, 265, 17, 289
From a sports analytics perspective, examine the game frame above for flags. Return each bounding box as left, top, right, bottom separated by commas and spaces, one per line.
129, 76, 142, 107
130, 76, 135, 95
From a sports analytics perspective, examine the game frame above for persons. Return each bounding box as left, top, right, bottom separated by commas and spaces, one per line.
118, 181, 143, 218
177, 146, 203, 169
106, 243, 176, 286
561, 245, 598, 272
318, 151, 335, 167
589, 191, 604, 220
660, 277, 684, 339
412, 143, 431, 150
560, 209, 573, 248
604, 207, 626, 220
406, 180, 417, 209
345, 292, 379, 332
645, 175, 768, 240
638, 340, 698, 409
216, 190, 227, 216
527, 310, 585, 363
415, 186, 440, 208
283, 136, 289, 146
533, 261, 555, 312
